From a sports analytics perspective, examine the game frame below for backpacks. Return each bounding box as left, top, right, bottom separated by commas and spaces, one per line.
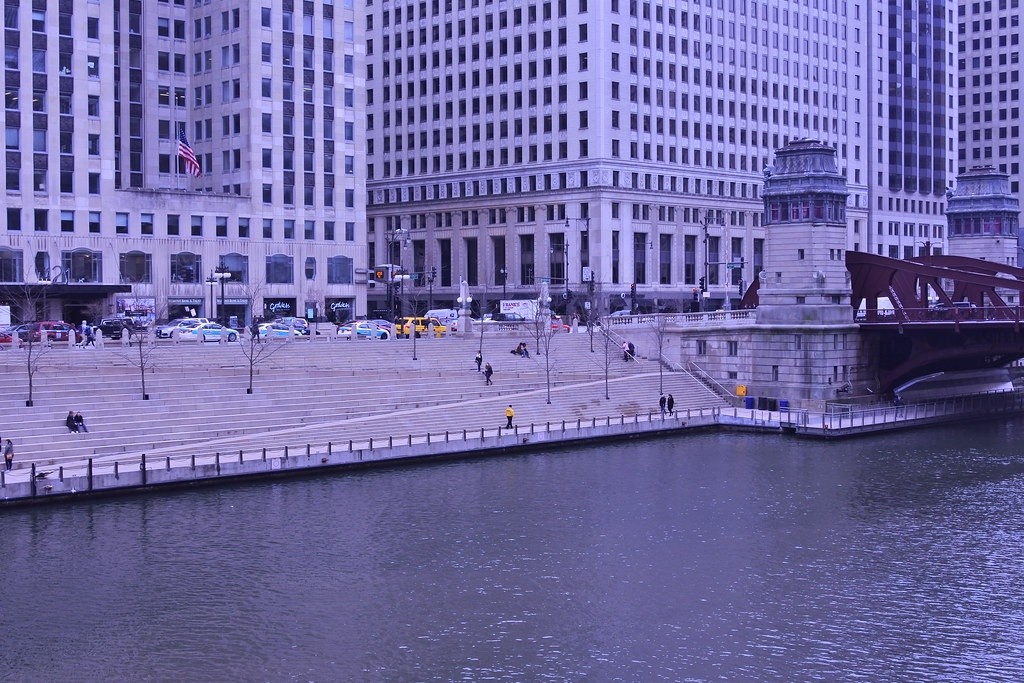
85, 328, 90, 336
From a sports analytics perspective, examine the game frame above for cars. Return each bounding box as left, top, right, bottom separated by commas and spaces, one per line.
0, 331, 22, 349
172, 322, 240, 344
492, 310, 632, 333
395, 317, 447, 339
6, 325, 32, 342
29, 321, 83, 346
156, 318, 209, 338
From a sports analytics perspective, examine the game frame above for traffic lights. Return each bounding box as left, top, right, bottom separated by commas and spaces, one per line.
694, 294, 698, 300
738, 280, 743, 295
374, 267, 388, 282
699, 277, 704, 289
590, 271, 595, 291
631, 283, 636, 298
431, 267, 437, 278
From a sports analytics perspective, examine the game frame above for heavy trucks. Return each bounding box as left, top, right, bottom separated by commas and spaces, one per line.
500, 299, 551, 321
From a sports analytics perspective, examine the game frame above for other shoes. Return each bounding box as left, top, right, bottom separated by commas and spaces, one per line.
76, 431, 78, 433
71, 431, 75, 433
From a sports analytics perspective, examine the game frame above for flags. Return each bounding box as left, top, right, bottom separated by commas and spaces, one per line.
178, 127, 200, 176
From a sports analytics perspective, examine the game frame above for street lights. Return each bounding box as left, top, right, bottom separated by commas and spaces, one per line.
391, 217, 590, 318
214, 266, 231, 327
206, 277, 218, 321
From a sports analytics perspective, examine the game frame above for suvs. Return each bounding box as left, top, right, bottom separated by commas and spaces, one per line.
80, 318, 137, 340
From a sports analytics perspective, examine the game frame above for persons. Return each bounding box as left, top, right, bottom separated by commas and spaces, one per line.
659, 393, 667, 415
505, 405, 514, 429
4, 439, 15, 472
621, 341, 635, 361
667, 394, 674, 416
485, 362, 493, 385
67, 322, 95, 348
475, 350, 482, 372
120, 321, 133, 347
516, 342, 530, 358
66, 411, 88, 434
251, 318, 260, 343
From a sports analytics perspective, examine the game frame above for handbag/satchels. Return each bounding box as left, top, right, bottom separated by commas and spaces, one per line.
7, 453, 12, 460
